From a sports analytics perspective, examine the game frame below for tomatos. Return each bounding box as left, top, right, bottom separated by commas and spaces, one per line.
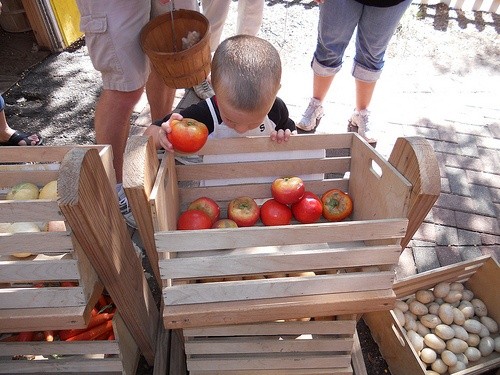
176, 176, 352, 229
166, 118, 208, 154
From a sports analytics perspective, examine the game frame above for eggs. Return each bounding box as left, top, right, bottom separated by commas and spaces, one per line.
392, 281, 500, 375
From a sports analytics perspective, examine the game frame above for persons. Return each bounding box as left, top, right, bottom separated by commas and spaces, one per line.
75, 0, 212, 230
296, 0, 412, 144
0, 95, 43, 146
192, 0, 264, 100
143, 34, 298, 187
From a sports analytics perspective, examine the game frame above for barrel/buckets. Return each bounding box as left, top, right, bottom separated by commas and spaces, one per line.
140, 0, 211, 90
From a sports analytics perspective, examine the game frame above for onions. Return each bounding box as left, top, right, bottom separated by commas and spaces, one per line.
7, 181, 68, 258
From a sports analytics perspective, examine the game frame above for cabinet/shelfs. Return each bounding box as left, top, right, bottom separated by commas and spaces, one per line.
0, 147, 169, 375
122, 135, 442, 375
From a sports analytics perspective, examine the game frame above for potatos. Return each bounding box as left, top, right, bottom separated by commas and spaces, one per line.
197, 269, 316, 322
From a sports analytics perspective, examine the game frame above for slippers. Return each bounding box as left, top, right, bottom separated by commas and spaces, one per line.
0, 128, 42, 147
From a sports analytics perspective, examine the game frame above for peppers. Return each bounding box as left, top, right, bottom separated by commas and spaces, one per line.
0, 281, 116, 343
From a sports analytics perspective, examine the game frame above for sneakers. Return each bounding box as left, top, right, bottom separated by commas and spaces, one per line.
115, 186, 139, 229
192, 76, 215, 100
295, 97, 325, 132
348, 107, 377, 145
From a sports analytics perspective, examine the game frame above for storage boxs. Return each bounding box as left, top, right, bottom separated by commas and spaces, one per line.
0, 146, 118, 308
180, 311, 357, 371
151, 130, 413, 305
363, 254, 500, 375
0, 310, 139, 375
